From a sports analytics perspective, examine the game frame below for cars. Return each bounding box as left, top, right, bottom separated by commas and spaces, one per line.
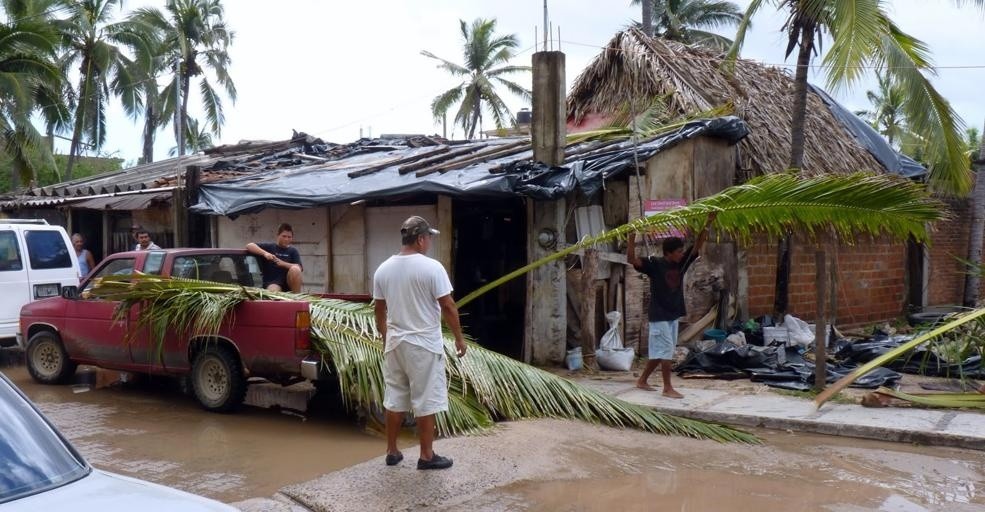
0, 368, 240, 512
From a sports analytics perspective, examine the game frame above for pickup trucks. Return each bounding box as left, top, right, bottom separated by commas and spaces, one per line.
15, 248, 387, 413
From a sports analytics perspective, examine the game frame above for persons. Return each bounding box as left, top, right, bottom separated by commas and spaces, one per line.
626, 210, 718, 399
71, 232, 96, 276
129, 224, 143, 251
244, 222, 304, 292
372, 214, 468, 471
135, 229, 161, 250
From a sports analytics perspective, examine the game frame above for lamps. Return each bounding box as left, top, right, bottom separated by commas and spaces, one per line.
534, 227, 557, 248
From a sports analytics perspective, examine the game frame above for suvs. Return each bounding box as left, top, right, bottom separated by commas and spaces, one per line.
0, 218, 80, 346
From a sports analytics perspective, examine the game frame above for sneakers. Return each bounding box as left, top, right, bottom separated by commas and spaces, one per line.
417, 451, 453, 469
386, 451, 402, 465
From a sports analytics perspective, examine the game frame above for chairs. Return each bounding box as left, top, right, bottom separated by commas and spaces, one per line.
207, 270, 233, 285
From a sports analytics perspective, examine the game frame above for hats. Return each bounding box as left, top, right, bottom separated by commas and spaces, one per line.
401, 216, 440, 237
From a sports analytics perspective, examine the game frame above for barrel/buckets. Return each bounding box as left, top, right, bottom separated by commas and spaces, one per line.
705, 329, 727, 342
762, 326, 788, 345
